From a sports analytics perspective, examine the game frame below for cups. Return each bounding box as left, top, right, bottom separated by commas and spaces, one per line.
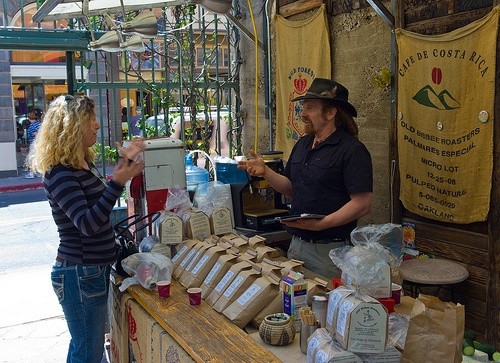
312, 294, 328, 328
391, 282, 402, 306
301, 320, 321, 354
157, 281, 171, 298
187, 288, 202, 306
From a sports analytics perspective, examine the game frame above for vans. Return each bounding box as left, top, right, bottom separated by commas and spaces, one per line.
137, 112, 236, 171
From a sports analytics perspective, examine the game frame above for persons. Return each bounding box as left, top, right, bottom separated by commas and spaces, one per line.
25, 93, 146, 363
24, 112, 44, 181
236, 76, 374, 278
16, 117, 32, 152
122, 105, 143, 139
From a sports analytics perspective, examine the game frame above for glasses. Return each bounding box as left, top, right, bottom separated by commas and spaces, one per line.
64, 93, 74, 102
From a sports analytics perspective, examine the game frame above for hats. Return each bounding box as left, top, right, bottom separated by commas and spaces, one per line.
291, 78, 357, 117
136, 106, 141, 110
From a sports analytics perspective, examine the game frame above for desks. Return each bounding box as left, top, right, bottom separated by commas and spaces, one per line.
127, 277, 282, 363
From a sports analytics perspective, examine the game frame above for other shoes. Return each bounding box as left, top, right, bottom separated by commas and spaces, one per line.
35, 173, 42, 178
24, 174, 34, 178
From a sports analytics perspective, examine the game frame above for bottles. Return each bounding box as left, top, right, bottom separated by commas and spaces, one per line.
185, 154, 210, 190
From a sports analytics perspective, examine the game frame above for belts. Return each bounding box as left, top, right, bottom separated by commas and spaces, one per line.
301, 237, 351, 244
56, 256, 78, 265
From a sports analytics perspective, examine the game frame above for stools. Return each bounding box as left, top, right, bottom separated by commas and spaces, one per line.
399, 258, 469, 308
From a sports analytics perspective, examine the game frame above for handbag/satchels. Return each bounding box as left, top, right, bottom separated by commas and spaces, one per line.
111, 211, 161, 277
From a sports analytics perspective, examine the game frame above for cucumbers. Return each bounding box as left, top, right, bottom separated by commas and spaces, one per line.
462, 329, 500, 362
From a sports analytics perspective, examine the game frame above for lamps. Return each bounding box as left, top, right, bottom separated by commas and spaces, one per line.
86, 0, 232, 53
